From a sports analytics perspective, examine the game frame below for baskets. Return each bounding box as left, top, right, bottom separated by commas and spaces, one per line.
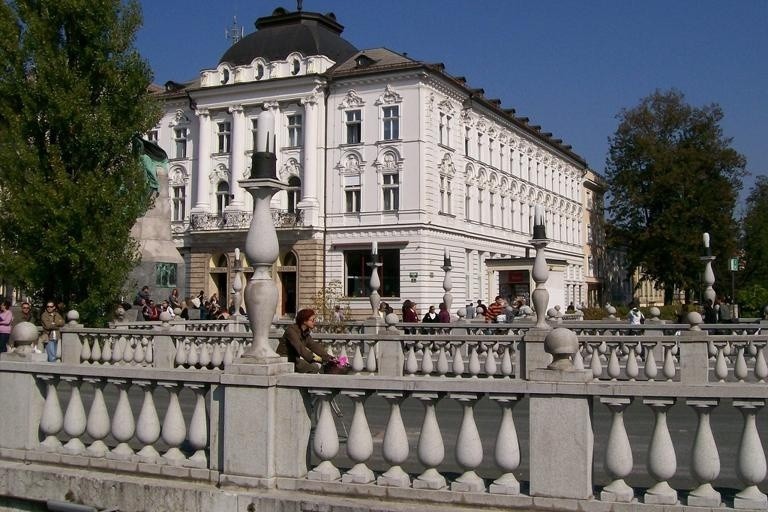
330, 360, 352, 374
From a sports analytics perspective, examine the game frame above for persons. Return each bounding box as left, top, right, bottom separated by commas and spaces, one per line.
14, 303, 35, 326
41, 301, 65, 363
334, 305, 345, 323
275, 309, 333, 374
567, 301, 574, 310
674, 304, 689, 324
466, 296, 503, 323
378, 300, 450, 323
627, 301, 646, 362
0, 301, 13, 353
701, 299, 731, 324
133, 286, 245, 320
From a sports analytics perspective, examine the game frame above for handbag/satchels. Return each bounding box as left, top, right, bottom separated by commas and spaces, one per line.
191, 297, 201, 308
39, 333, 49, 344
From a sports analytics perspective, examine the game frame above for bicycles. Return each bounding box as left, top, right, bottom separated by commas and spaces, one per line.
308, 357, 366, 445
